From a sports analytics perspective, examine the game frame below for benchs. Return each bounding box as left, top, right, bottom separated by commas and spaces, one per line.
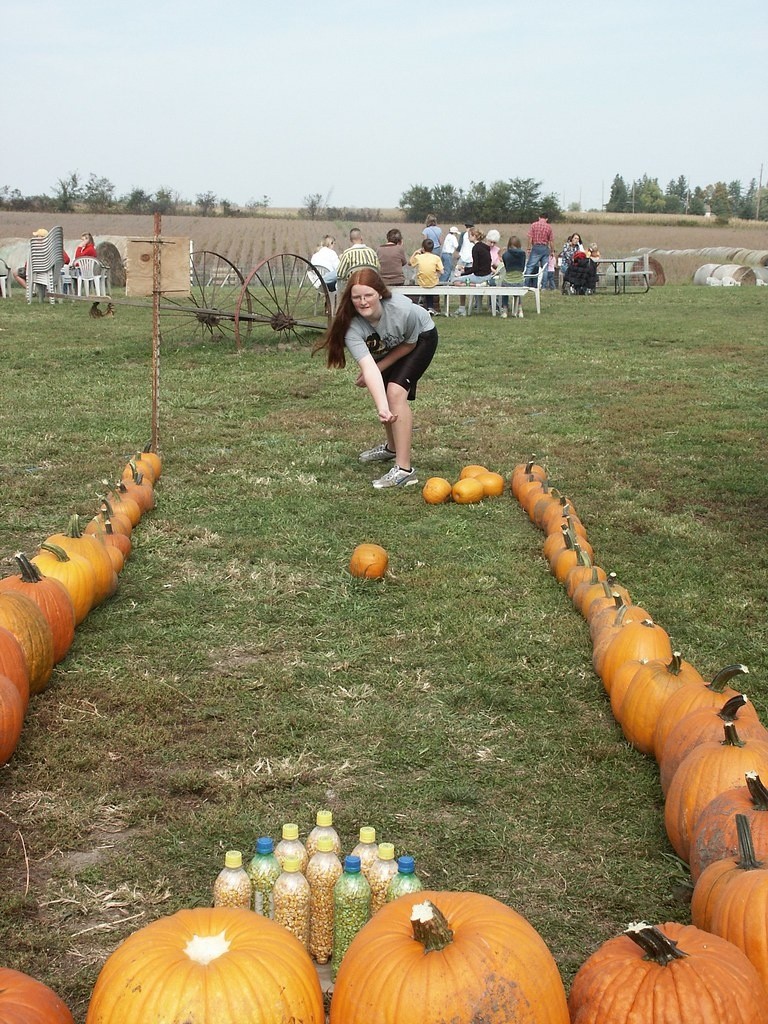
386, 286, 529, 316
596, 271, 653, 295
559, 272, 606, 294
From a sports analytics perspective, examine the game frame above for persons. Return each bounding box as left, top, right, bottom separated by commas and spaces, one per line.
525, 215, 554, 290
13, 229, 70, 297
501, 236, 526, 318
311, 268, 439, 489
75, 233, 97, 295
561, 233, 601, 293
547, 250, 556, 290
308, 235, 340, 314
376, 228, 406, 286
408, 239, 445, 316
423, 214, 442, 255
336, 227, 380, 290
440, 221, 503, 316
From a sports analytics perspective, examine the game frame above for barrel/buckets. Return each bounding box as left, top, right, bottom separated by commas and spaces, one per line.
733, 249, 768, 283
692, 263, 756, 287
636, 247, 743, 279
606, 256, 665, 286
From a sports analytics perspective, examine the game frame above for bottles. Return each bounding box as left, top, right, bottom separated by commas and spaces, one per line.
466, 275, 470, 287
309, 811, 341, 860
306, 837, 343, 964
417, 249, 424, 255
275, 823, 308, 873
213, 850, 252, 911
274, 857, 310, 959
349, 826, 379, 876
247, 838, 280, 924
331, 857, 371, 983
370, 843, 399, 915
387, 857, 424, 903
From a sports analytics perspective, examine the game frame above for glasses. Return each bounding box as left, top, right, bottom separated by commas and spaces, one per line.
351, 291, 380, 303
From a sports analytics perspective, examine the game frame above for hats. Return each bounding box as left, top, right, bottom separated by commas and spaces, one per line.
465, 220, 475, 227
487, 230, 500, 243
448, 227, 461, 235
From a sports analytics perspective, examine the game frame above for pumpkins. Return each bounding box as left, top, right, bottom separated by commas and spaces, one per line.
0, 443, 768, 1024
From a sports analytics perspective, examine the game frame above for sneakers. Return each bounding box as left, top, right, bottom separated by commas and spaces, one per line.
372, 465, 418, 489
357, 444, 398, 463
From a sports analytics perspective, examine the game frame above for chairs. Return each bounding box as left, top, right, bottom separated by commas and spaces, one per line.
307, 265, 337, 318
0, 259, 12, 298
25, 225, 111, 307
467, 262, 548, 317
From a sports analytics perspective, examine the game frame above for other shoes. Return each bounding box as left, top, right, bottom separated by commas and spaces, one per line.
495, 306, 499, 312
500, 312, 507, 318
518, 313, 523, 318
427, 308, 441, 316
474, 306, 477, 310
451, 309, 467, 317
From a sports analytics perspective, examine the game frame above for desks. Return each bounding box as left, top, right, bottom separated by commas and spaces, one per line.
594, 257, 640, 294
